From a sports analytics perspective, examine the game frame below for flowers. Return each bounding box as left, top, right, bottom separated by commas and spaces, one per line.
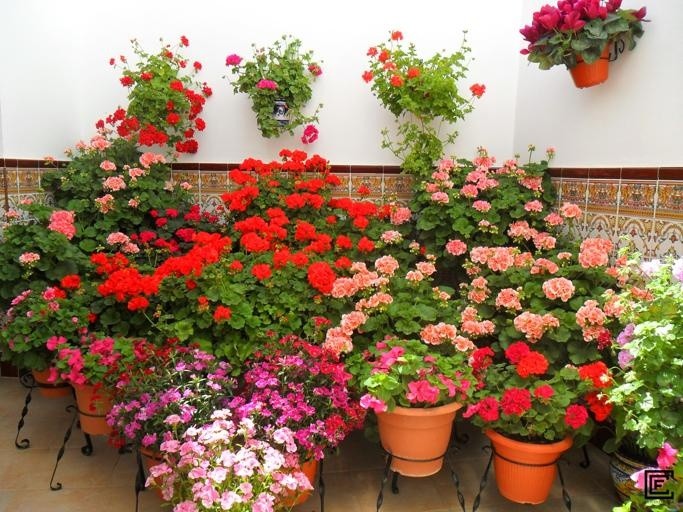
361, 30, 485, 179
221, 34, 323, 145
95, 35, 211, 162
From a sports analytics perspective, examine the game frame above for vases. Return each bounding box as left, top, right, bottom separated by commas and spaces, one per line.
563, 40, 612, 88
272, 99, 291, 121
519, 1, 651, 71
